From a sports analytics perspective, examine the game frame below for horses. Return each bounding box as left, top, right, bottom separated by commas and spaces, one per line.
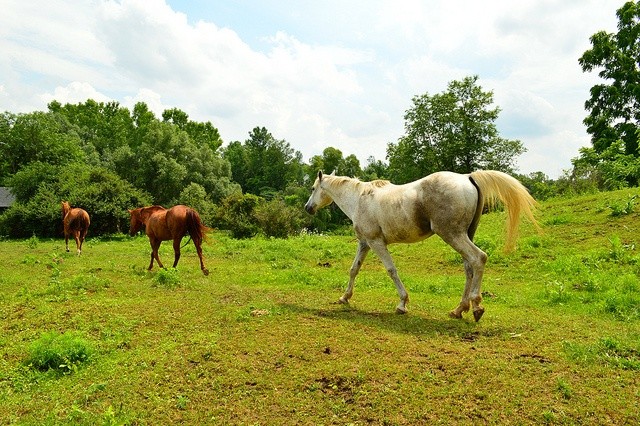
61, 201, 91, 256
127, 204, 211, 275
304, 169, 544, 323
